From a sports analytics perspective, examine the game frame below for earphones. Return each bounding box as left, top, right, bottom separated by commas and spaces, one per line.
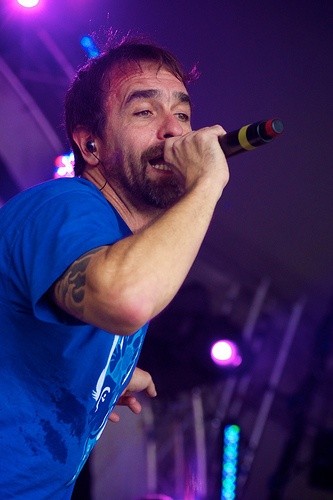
87, 141, 96, 153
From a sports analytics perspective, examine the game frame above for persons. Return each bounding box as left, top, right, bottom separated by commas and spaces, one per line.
1, 43, 231, 499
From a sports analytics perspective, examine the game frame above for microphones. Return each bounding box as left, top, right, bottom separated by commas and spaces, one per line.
216, 119, 284, 157
